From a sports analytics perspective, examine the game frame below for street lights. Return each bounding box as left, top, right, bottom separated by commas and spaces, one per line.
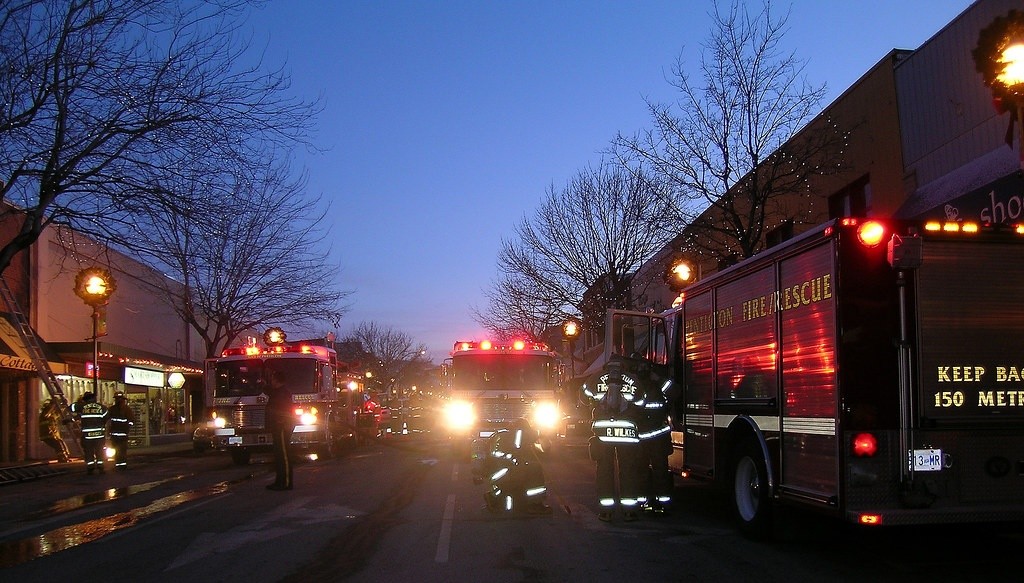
73, 267, 115, 404
563, 320, 582, 381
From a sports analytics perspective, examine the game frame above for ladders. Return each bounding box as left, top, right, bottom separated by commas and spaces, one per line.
0, 274, 85, 461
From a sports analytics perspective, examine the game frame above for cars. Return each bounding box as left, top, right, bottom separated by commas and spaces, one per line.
191, 424, 216, 453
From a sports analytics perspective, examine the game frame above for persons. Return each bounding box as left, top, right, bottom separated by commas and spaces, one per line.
77, 391, 135, 476
38, 398, 73, 462
268, 356, 674, 521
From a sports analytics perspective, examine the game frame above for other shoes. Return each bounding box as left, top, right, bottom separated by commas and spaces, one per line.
638, 502, 671, 519
266, 480, 293, 491
624, 510, 638, 520
598, 510, 613, 521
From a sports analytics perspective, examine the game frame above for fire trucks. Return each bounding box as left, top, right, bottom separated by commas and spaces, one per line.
569, 214, 1024, 540
204, 318, 598, 468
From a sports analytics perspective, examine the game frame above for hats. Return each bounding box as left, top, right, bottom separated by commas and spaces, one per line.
113, 391, 125, 398
